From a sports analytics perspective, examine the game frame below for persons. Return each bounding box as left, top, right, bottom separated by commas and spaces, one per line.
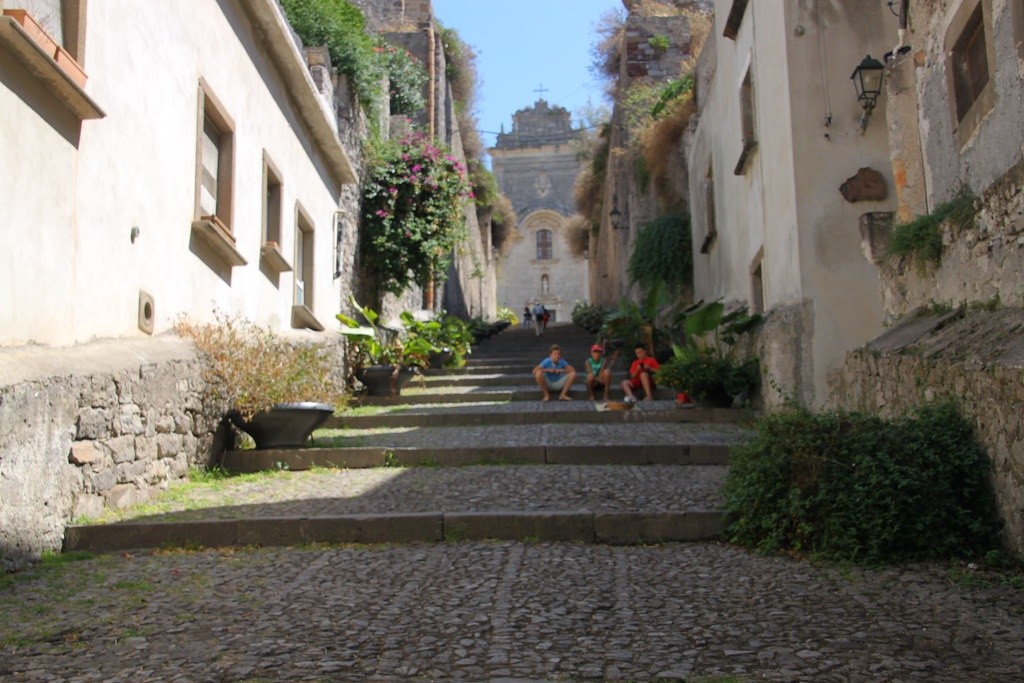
533, 344, 576, 400
523, 301, 550, 337
584, 344, 611, 400
622, 344, 659, 402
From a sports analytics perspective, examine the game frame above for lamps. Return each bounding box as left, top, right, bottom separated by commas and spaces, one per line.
850, 54, 885, 136
609, 205, 629, 230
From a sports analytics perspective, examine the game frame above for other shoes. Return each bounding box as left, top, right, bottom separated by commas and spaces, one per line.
603, 397, 611, 402
624, 396, 636, 402
588, 396, 595, 403
558, 395, 572, 401
642, 397, 654, 403
543, 396, 551, 402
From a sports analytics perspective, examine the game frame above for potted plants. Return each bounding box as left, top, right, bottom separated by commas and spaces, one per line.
673, 293, 771, 403
171, 296, 339, 451
336, 289, 436, 395
654, 349, 760, 411
399, 308, 518, 371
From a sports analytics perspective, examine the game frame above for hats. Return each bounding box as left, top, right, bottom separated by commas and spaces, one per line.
592, 345, 602, 352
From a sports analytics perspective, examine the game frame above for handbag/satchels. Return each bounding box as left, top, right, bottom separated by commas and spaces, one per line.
536, 314, 544, 321
